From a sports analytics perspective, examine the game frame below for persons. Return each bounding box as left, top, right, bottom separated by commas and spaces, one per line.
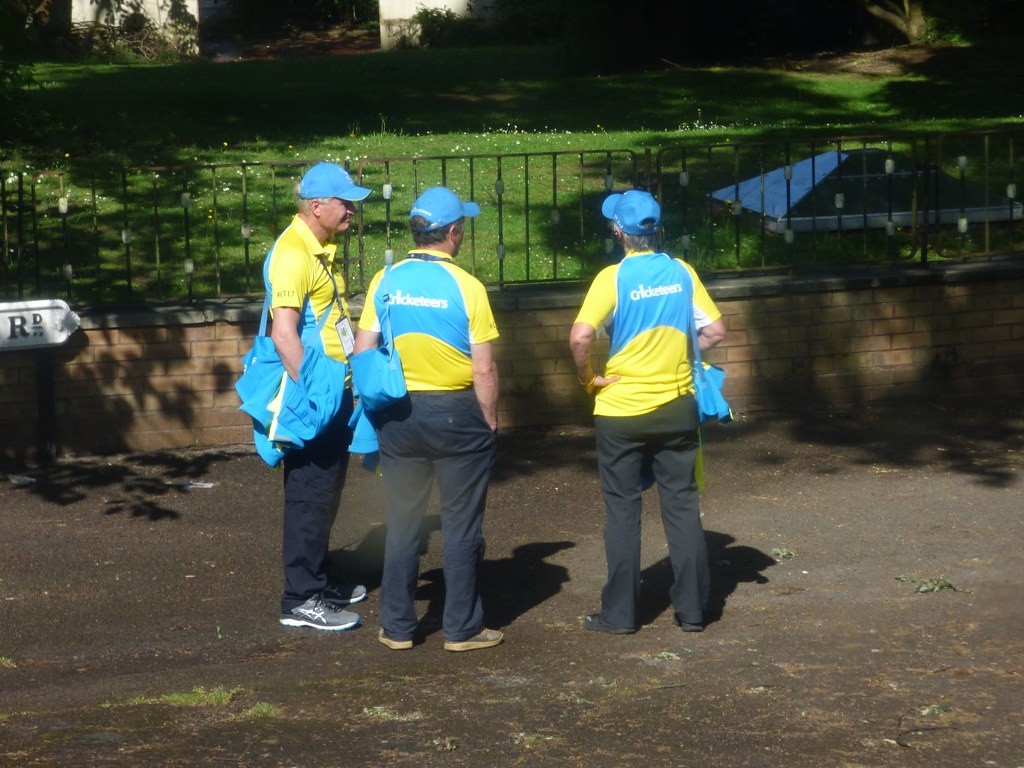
353, 186, 504, 649
569, 190, 727, 635
263, 163, 366, 632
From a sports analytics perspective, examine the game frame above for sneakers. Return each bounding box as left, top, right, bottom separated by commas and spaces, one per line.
585, 613, 635, 634
673, 614, 703, 632
280, 595, 359, 630
378, 625, 413, 650
444, 623, 503, 651
319, 580, 367, 606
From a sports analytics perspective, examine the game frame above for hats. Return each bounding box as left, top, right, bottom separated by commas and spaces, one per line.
602, 190, 661, 237
299, 162, 372, 201
408, 186, 481, 234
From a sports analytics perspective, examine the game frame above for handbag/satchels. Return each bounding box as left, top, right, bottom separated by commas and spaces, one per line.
347, 264, 407, 412
673, 259, 733, 423
